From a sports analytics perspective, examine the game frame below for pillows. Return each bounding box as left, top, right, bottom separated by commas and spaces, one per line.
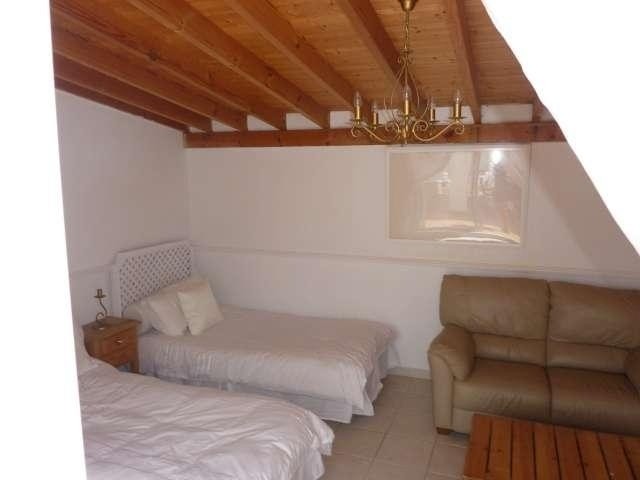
124, 273, 226, 335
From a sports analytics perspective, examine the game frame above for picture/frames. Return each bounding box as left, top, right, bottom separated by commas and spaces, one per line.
385, 142, 531, 250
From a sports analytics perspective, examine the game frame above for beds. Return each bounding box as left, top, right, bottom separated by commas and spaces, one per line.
107, 241, 398, 423
72, 321, 335, 480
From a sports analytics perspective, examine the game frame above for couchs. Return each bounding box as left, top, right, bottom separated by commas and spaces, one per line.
424, 272, 640, 438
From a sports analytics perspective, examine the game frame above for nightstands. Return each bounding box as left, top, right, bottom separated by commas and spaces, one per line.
83, 316, 142, 374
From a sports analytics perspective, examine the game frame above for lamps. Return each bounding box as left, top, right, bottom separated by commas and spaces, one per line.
349, 0, 467, 147
